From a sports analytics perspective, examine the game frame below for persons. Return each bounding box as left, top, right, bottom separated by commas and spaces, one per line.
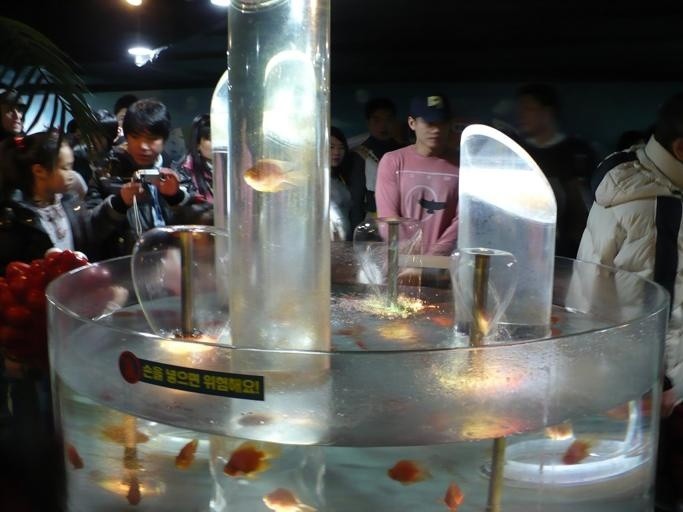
566, 94, 682, 473
375, 87, 459, 288
513, 85, 599, 258
328, 124, 367, 241
0, 89, 214, 466
355, 92, 403, 220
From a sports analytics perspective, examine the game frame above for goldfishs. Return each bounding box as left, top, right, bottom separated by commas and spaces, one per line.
533, 411, 597, 466
387, 459, 470, 512
67, 446, 84, 470
462, 414, 526, 439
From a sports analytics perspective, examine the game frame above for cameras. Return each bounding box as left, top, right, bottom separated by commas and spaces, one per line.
135, 169, 160, 184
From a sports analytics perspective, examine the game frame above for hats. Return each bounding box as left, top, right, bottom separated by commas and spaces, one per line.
406, 90, 453, 123
